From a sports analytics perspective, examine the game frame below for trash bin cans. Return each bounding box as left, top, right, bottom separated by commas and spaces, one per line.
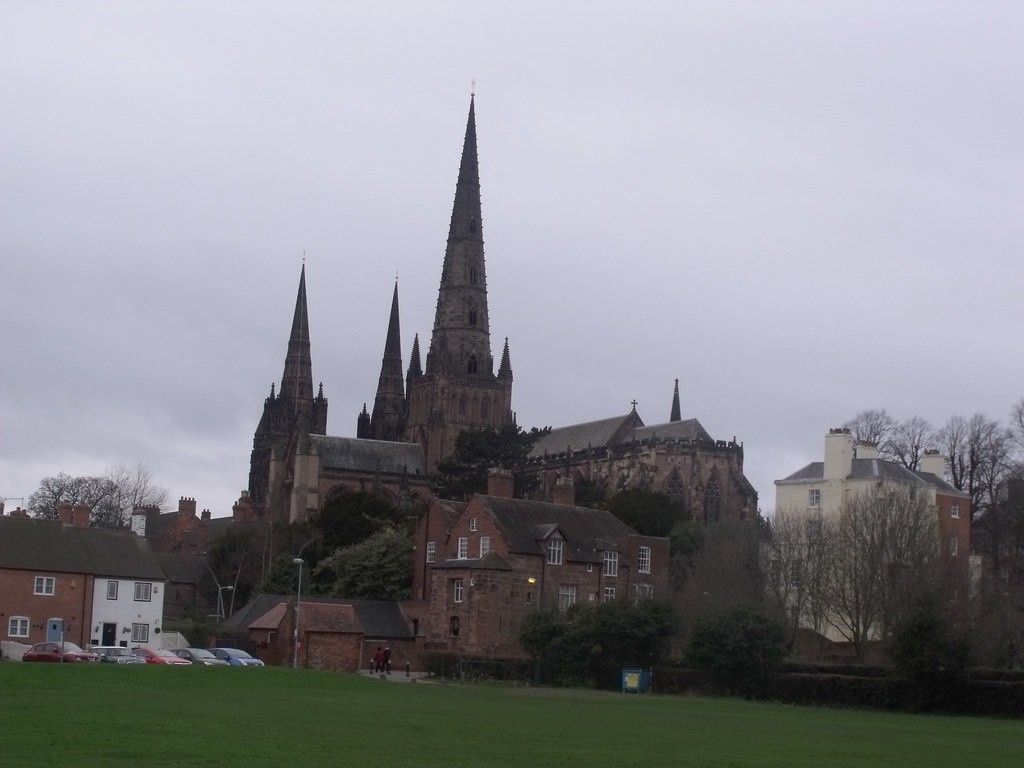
621, 667, 649, 696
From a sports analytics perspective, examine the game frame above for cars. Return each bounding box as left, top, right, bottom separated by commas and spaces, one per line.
173, 648, 230, 665
22, 641, 100, 663
89, 646, 148, 665
207, 647, 264, 667
136, 647, 194, 666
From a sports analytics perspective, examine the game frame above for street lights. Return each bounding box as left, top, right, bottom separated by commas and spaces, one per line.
291, 551, 304, 668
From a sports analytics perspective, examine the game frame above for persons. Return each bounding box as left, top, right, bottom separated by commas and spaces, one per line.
382, 646, 391, 673
374, 647, 385, 672
